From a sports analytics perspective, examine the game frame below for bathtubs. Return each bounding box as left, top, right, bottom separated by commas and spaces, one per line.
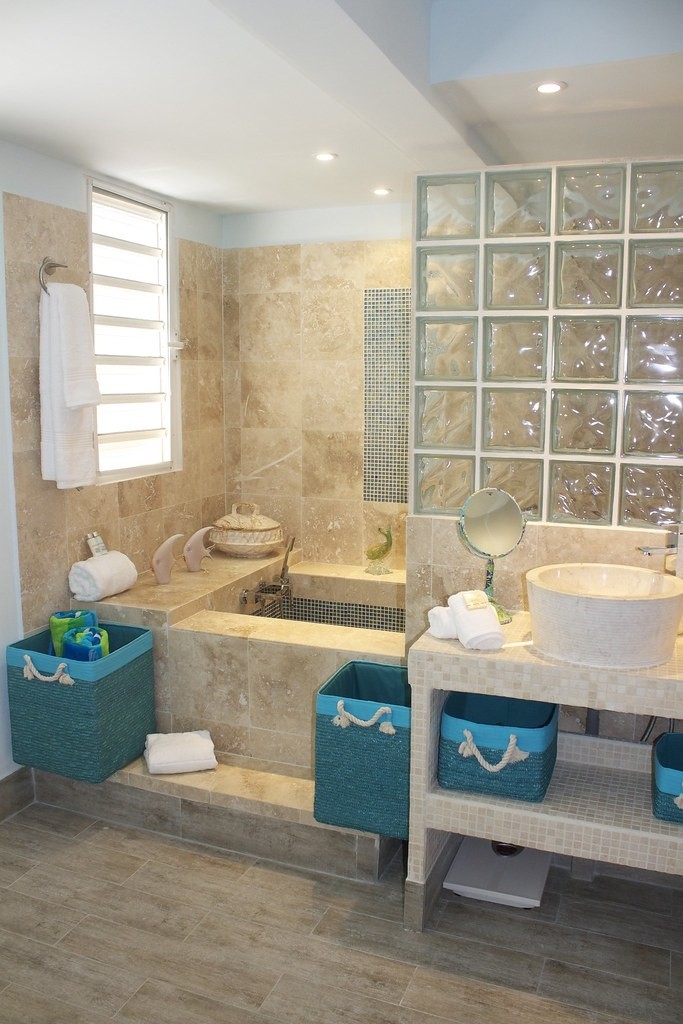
170, 560, 406, 782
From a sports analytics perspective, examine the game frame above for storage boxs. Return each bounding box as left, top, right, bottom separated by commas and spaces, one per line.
439, 691, 558, 803
313, 659, 413, 843
6, 618, 155, 783
652, 732, 683, 824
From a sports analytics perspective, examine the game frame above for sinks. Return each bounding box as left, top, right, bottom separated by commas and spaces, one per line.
524, 563, 683, 668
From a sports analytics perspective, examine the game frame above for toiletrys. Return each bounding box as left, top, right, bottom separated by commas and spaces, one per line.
86, 531, 107, 555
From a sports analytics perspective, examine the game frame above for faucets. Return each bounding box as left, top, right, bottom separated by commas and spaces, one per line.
238, 536, 296, 604
636, 523, 682, 558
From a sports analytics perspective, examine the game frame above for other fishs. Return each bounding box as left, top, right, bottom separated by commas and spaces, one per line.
149, 524, 215, 585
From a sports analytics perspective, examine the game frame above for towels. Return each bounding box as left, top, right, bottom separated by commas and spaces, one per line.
142, 730, 218, 775
428, 602, 498, 639
49, 608, 108, 662
448, 591, 503, 650
39, 280, 101, 489
66, 552, 138, 601
